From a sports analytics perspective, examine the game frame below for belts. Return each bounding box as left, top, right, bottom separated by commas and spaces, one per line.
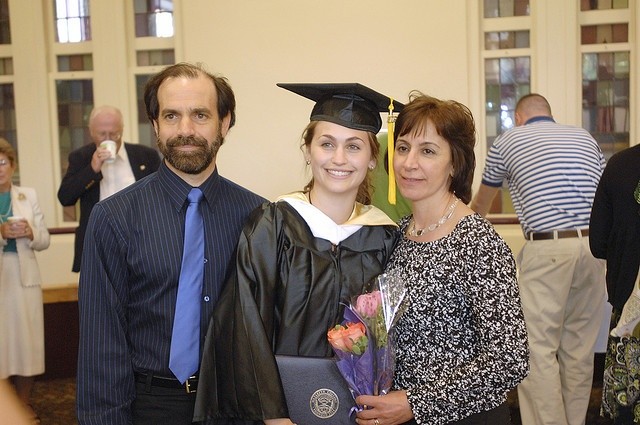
526, 228, 589, 240
134, 372, 198, 393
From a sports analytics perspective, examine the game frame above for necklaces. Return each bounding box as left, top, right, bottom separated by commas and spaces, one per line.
0, 198, 12, 218
405, 198, 463, 238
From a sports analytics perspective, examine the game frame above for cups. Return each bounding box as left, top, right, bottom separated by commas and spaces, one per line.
99, 140, 117, 163
7, 216, 24, 227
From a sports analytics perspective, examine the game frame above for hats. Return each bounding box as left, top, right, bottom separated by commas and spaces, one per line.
276, 82, 404, 206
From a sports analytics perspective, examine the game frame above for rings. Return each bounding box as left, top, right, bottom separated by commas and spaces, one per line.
97, 154, 100, 157
373, 418, 380, 425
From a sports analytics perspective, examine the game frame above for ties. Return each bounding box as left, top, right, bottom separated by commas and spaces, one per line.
169, 188, 207, 386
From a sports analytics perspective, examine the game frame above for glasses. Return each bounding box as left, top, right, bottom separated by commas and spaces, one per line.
0, 160, 11, 166
90, 131, 123, 141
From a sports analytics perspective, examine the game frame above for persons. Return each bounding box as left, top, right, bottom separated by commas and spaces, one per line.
59, 102, 161, 272
1, 138, 51, 424
192, 82, 408, 425
472, 92, 606, 425
355, 88, 529, 425
77, 62, 274, 425
590, 141, 640, 421
